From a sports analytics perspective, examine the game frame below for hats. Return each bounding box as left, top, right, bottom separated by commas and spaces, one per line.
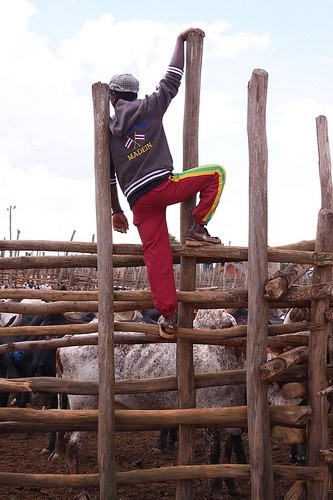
109, 74, 139, 92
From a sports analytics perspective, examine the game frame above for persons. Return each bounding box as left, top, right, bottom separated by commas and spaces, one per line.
109, 28, 227, 339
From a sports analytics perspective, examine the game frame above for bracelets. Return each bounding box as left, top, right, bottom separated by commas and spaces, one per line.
110, 209, 125, 215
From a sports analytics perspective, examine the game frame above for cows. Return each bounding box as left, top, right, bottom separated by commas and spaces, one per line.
1, 280, 311, 500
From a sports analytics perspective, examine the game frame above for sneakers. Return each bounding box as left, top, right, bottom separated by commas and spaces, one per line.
186, 229, 223, 247
158, 315, 177, 338
